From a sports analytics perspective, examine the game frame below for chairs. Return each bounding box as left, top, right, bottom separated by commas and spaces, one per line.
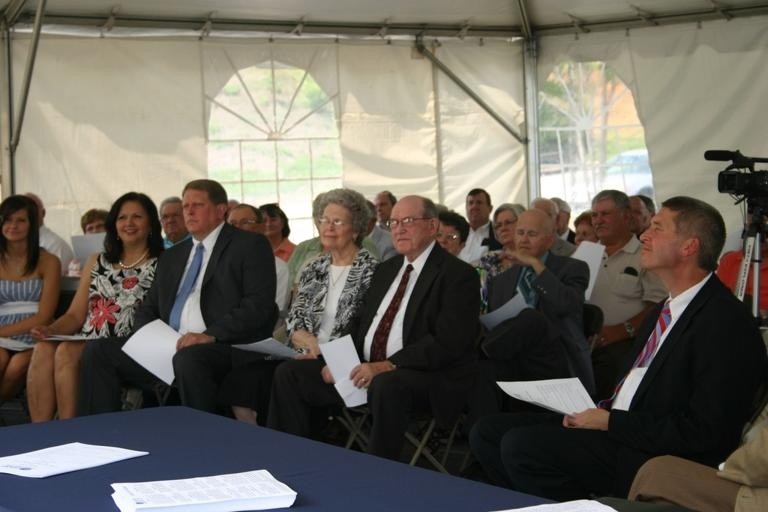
334, 321, 485, 479
580, 301, 605, 358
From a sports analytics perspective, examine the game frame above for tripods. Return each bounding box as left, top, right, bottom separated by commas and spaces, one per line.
733, 227, 768, 318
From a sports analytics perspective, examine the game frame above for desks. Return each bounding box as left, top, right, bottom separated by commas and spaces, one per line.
3, 404, 564, 506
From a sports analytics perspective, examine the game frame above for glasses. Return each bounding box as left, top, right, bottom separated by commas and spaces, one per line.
436, 230, 464, 242
317, 215, 356, 228
384, 216, 432, 228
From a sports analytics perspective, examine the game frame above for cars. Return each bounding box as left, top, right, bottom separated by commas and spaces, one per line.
573, 148, 655, 214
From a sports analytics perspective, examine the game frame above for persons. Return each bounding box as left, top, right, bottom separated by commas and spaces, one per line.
1, 179, 768, 512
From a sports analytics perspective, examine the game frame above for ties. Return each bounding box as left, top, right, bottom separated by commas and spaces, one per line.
167, 241, 207, 333
365, 264, 416, 364
595, 300, 672, 411
517, 267, 539, 309
